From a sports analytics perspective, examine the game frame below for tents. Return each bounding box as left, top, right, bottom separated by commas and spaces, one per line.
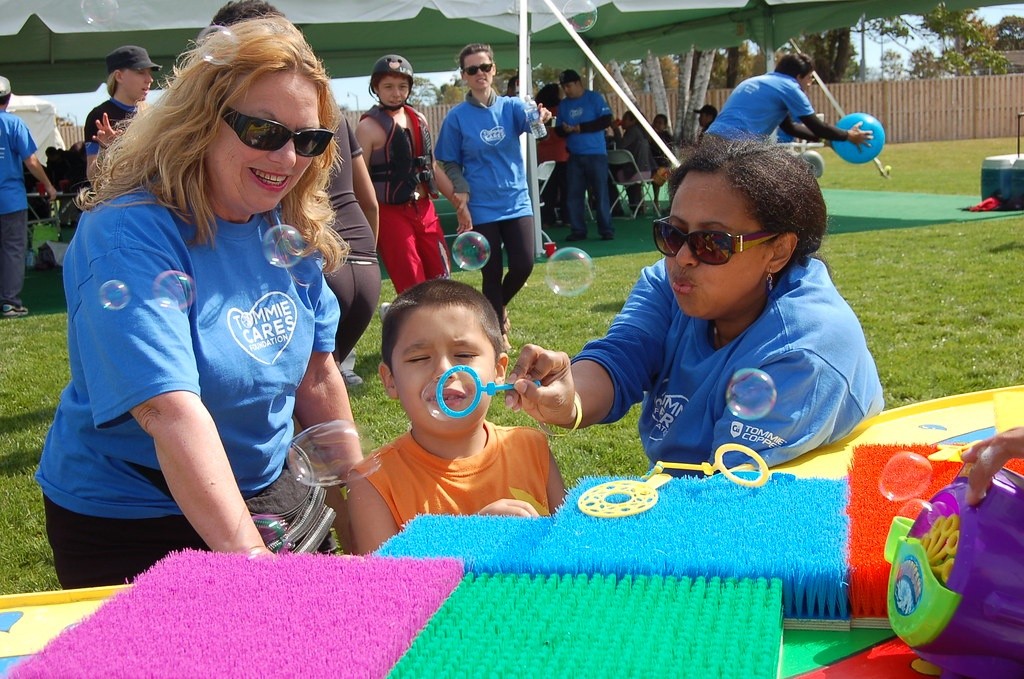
0, 0, 1024, 283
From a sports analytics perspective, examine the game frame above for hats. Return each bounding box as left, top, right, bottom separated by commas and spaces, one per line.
106, 46, 162, 75
558, 69, 580, 85
693, 104, 717, 117
0, 76, 11, 96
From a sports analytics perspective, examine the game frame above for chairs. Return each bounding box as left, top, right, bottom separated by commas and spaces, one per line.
538, 159, 558, 250
609, 149, 662, 222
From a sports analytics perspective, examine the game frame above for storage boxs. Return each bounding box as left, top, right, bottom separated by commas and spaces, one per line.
981, 153, 1024, 209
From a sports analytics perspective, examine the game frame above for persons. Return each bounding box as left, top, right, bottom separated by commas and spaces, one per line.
498, 132, 886, 477
1, 43, 879, 383
34, 13, 365, 589
346, 280, 567, 559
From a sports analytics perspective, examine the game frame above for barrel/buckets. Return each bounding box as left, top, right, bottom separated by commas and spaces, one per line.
26, 249, 36, 269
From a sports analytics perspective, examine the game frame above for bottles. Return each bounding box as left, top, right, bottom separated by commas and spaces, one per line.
524, 95, 547, 140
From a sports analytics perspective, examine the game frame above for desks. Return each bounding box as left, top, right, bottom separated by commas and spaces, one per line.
27, 192, 96, 250
0, 387, 1024, 679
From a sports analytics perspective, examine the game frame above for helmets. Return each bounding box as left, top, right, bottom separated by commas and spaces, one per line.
371, 55, 414, 87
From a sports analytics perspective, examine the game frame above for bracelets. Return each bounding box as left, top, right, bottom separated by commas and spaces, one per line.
538, 396, 582, 438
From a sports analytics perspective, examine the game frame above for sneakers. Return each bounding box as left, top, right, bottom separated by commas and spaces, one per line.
0, 304, 29, 316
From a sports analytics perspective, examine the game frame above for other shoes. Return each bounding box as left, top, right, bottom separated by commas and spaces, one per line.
601, 233, 615, 240
542, 220, 554, 228
562, 221, 571, 227
564, 234, 587, 241
613, 209, 624, 216
341, 369, 363, 386
378, 302, 390, 321
633, 210, 646, 216
502, 315, 516, 357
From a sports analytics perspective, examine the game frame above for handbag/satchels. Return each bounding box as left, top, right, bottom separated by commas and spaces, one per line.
25, 222, 69, 271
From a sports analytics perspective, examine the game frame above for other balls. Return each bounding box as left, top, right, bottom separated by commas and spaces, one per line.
830, 112, 886, 164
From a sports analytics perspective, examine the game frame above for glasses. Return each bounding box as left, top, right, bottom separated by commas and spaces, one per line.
217, 103, 334, 157
807, 74, 815, 85
462, 63, 494, 76
652, 215, 779, 266
